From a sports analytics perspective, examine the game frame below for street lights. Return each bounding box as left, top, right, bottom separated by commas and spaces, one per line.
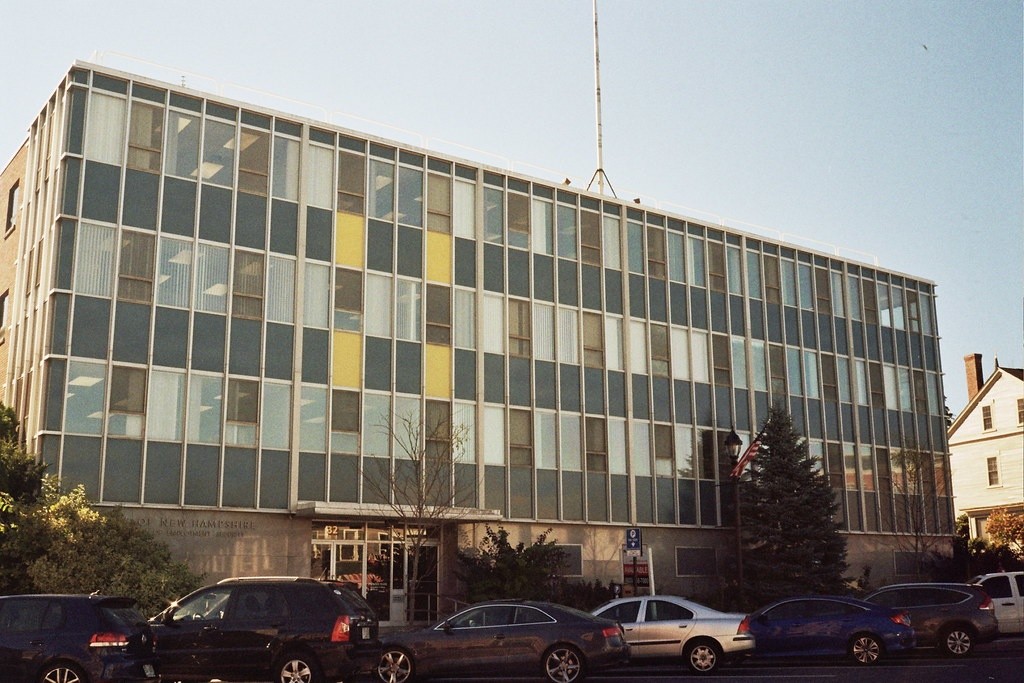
723, 425, 747, 614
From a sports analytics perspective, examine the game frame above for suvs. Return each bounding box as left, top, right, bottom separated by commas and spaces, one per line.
969, 572, 1023, 635
860, 582, 999, 660
149, 575, 380, 683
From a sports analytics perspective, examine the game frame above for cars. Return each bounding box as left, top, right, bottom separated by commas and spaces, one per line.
749, 597, 915, 667
0, 590, 155, 683
378, 596, 628, 683
588, 594, 755, 674
